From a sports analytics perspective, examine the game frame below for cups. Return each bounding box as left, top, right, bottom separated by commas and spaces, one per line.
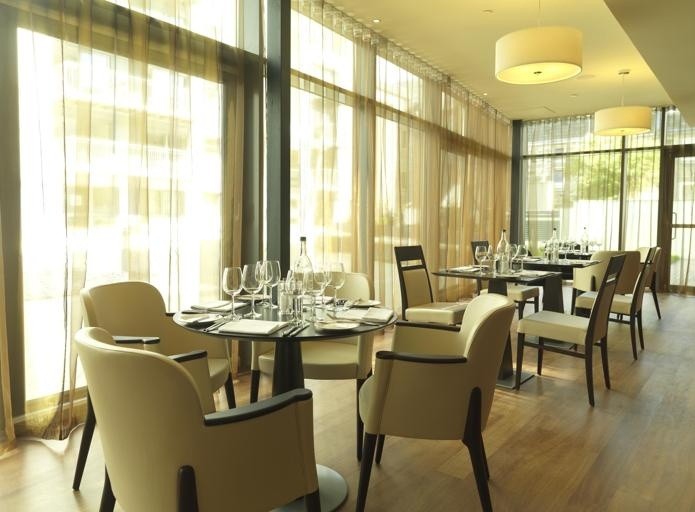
255, 261, 272, 307
326, 262, 345, 311
263, 260, 281, 310
474, 246, 487, 275
223, 267, 243, 321
518, 245, 528, 272
561, 247, 569, 263
303, 267, 326, 322
314, 265, 332, 309
505, 244, 517, 273
488, 245, 500, 276
242, 264, 263, 318
284, 270, 307, 325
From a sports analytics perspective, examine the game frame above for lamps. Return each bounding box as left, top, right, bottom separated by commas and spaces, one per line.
494, 27, 582, 86
594, 105, 651, 137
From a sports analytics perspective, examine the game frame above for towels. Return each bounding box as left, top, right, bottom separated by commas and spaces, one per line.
218, 318, 289, 334
363, 307, 394, 324
516, 270, 555, 278
449, 266, 480, 273
191, 298, 247, 314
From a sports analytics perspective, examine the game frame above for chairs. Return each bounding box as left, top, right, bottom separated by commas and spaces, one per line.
516, 254, 626, 408
472, 240, 539, 319
394, 246, 468, 326
73, 281, 235, 493
74, 328, 321, 512
250, 272, 374, 463
575, 246, 657, 360
355, 292, 515, 512
618, 246, 661, 322
570, 251, 641, 316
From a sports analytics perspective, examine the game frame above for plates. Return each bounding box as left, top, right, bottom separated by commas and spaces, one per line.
353, 299, 381, 307
178, 313, 222, 324
237, 294, 271, 301
315, 320, 359, 332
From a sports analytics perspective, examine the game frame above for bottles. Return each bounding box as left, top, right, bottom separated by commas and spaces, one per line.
581, 227, 589, 241
497, 229, 508, 254
293, 237, 313, 290
550, 228, 559, 243
